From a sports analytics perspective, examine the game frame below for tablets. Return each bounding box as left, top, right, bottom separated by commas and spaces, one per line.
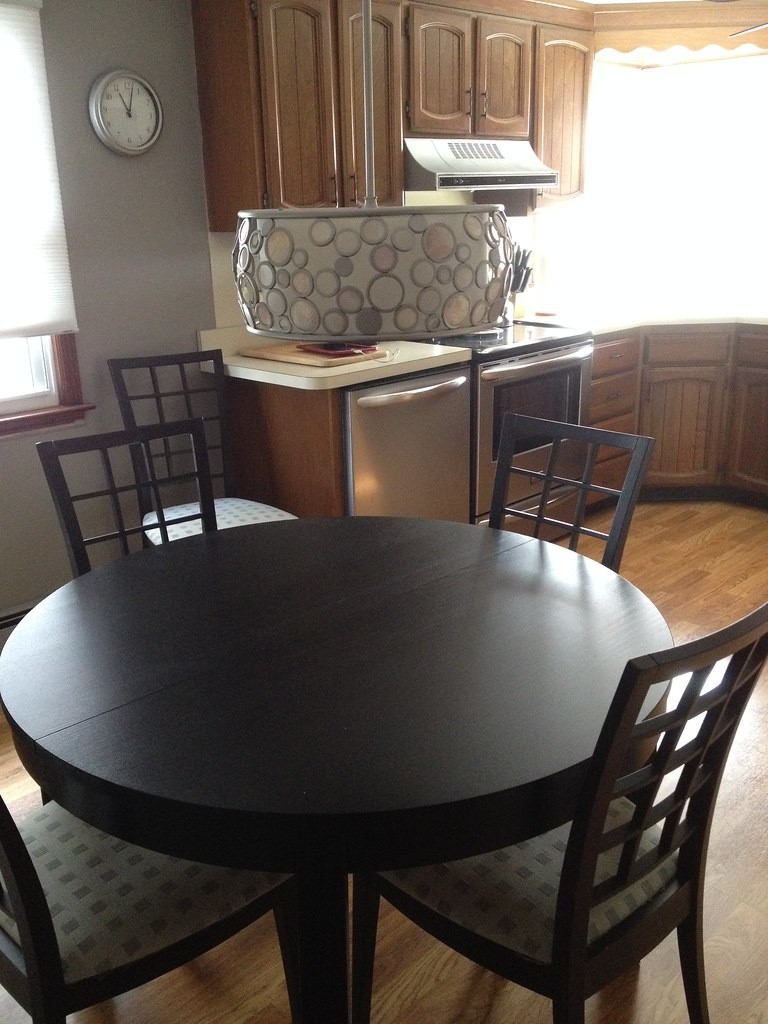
296, 342, 377, 355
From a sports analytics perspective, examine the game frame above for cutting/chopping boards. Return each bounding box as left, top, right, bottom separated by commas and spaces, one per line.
241, 343, 389, 367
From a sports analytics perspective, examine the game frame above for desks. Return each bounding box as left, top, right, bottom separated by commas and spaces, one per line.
0, 515, 673, 1024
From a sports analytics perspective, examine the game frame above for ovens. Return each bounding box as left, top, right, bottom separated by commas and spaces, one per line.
472, 340, 595, 543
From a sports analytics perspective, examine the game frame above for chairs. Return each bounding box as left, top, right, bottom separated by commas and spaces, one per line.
107, 349, 299, 550
349, 601, 768, 1024
487, 414, 656, 573
0, 794, 297, 1024
35, 416, 218, 580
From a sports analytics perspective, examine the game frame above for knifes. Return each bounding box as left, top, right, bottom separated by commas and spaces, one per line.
513, 244, 534, 292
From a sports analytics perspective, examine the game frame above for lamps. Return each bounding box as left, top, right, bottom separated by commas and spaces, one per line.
232, 0, 516, 340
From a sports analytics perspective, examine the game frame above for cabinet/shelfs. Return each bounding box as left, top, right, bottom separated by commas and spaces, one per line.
585, 322, 738, 518
404, 0, 535, 142
722, 323, 768, 504
191, 0, 405, 234
474, 0, 596, 216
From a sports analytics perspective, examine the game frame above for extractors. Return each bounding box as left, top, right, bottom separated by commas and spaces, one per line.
402, 137, 559, 191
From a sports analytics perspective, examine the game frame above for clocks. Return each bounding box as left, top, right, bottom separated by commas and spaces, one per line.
88, 69, 163, 157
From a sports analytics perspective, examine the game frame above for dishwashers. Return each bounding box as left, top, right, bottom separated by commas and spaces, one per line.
344, 363, 472, 526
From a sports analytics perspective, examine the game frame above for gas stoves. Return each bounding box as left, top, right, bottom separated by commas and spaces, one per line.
459, 326, 505, 343
